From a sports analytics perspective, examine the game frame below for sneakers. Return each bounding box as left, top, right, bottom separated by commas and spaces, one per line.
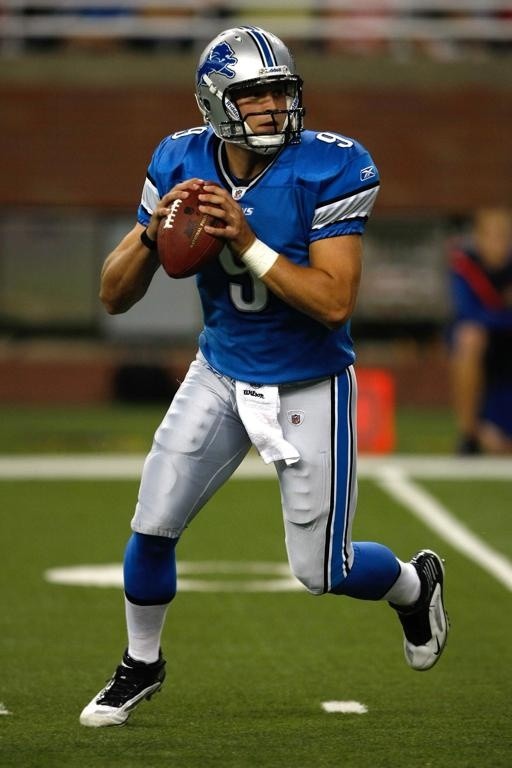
79, 645, 166, 727
388, 548, 449, 672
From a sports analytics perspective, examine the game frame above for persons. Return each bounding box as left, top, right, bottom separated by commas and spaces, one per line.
1, 0, 512, 63
72, 22, 455, 736
439, 198, 510, 461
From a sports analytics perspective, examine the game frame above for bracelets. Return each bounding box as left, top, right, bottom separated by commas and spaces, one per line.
138, 226, 159, 251
237, 237, 281, 279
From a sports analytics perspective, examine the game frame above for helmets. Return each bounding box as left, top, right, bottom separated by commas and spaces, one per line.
194, 26, 305, 156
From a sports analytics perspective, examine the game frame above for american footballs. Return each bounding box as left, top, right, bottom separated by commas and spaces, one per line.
157, 181, 226, 279
